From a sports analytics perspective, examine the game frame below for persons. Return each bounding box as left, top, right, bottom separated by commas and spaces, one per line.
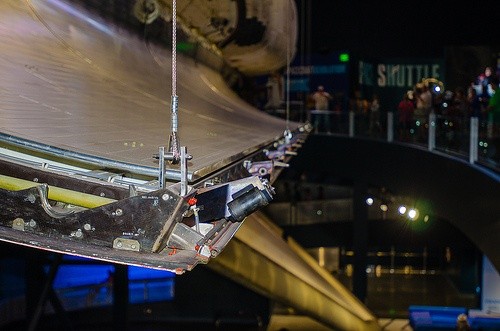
309, 86, 334, 134
350, 241, 374, 306
